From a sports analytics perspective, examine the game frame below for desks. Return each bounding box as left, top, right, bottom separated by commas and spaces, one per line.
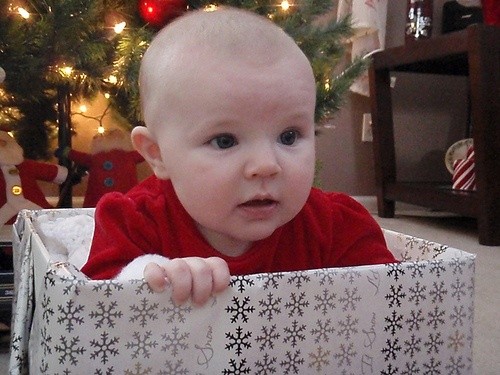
363, 24, 500, 249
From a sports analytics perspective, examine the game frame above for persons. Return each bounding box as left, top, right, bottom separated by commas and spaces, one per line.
80, 3, 400, 310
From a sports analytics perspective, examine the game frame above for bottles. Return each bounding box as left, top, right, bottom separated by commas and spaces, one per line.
405, 0, 433, 43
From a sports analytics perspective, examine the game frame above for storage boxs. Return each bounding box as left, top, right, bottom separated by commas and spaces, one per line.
7, 200, 476, 374
